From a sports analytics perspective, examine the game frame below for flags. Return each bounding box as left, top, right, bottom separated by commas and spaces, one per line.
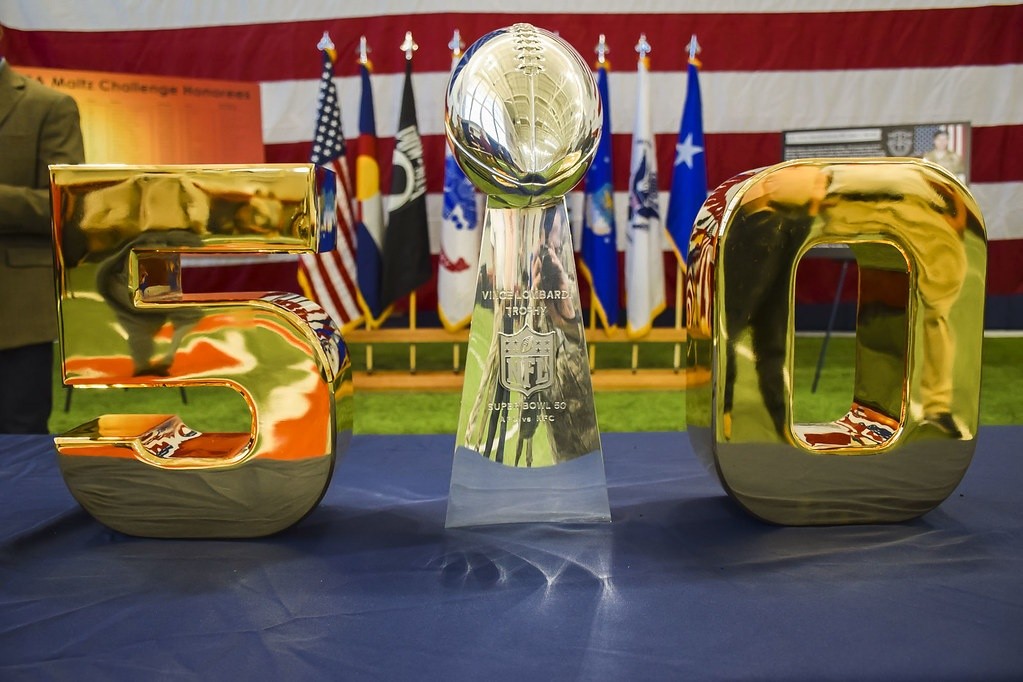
297, 48, 708, 338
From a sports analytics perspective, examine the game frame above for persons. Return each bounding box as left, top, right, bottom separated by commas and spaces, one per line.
922, 129, 961, 175
0, 57, 86, 433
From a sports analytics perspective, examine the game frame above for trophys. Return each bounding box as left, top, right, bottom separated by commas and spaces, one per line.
441, 22, 613, 527
